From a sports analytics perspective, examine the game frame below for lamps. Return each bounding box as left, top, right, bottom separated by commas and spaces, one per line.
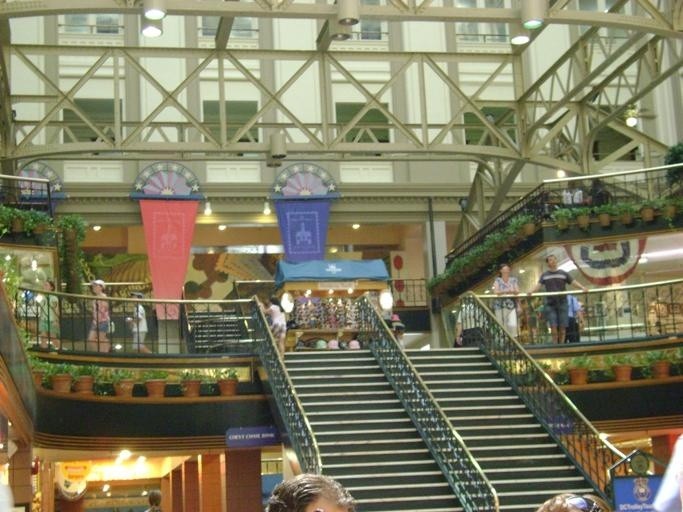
266, 135, 289, 170
323, 0, 364, 43
507, 0, 547, 51
135, 0, 172, 44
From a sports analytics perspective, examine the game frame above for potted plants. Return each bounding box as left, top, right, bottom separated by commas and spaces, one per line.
0, 202, 90, 299
504, 345, 681, 388
424, 190, 681, 297
16, 353, 245, 400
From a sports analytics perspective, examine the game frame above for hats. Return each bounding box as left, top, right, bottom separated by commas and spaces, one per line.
93, 280, 106, 290
131, 292, 144, 299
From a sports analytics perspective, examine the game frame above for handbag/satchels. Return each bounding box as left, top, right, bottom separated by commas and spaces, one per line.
108, 320, 115, 334
516, 302, 522, 316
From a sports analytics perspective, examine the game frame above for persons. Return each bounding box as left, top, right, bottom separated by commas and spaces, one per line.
122, 291, 152, 353
560, 180, 583, 207
265, 471, 357, 511
88, 280, 109, 352
455, 297, 484, 347
565, 289, 584, 344
35, 280, 61, 350
492, 263, 520, 344
527, 254, 589, 345
259, 296, 286, 362
536, 492, 608, 512
142, 490, 162, 511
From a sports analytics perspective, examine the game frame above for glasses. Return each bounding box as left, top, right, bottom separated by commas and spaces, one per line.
566, 495, 601, 512
